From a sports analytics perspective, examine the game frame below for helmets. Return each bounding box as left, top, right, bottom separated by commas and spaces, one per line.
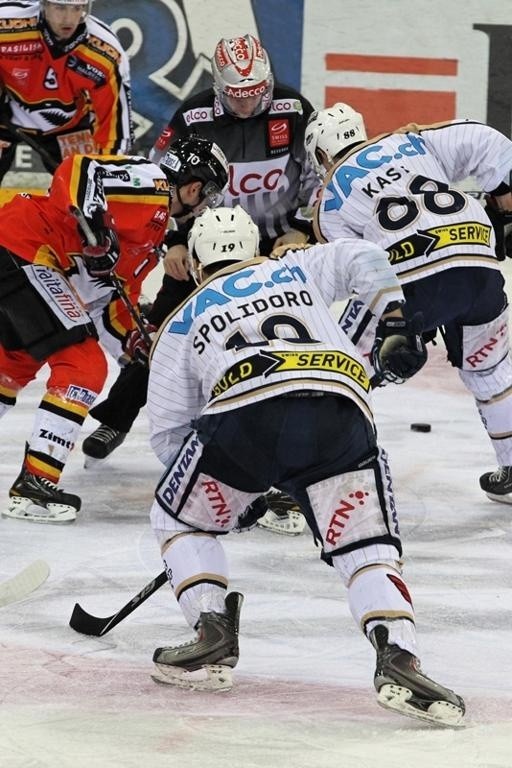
160, 133, 231, 209
211, 34, 275, 117
187, 205, 261, 286
303, 102, 367, 170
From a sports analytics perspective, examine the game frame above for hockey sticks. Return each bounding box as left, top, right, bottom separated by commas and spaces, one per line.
70, 374, 383, 637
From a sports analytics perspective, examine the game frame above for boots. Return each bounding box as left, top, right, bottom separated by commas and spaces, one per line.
150, 592, 245, 693
3, 467, 82, 523
480, 466, 512, 504
84, 422, 128, 470
369, 624, 466, 731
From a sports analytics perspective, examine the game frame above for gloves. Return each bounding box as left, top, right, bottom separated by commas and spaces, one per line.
84, 230, 120, 277
377, 317, 427, 379
123, 323, 158, 367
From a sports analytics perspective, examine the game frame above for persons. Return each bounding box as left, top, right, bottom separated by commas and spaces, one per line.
149, 206, 469, 730
1, 134, 230, 523
82, 32, 324, 469
249, 102, 511, 538
2, 0, 134, 186
